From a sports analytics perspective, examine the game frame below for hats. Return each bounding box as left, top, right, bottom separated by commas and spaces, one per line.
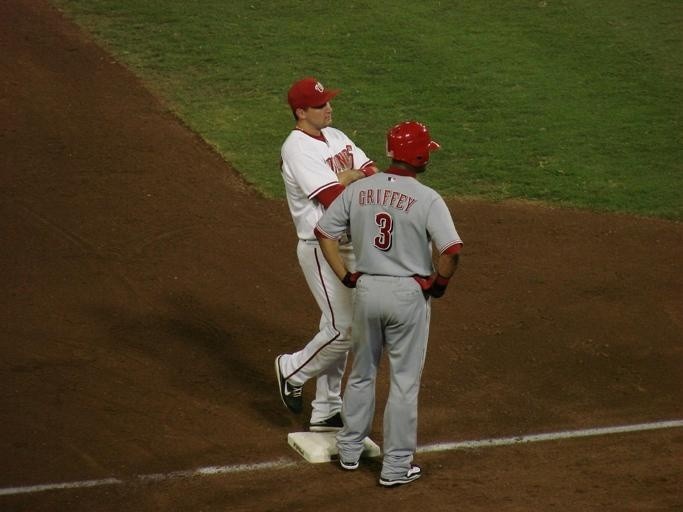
288, 79, 342, 112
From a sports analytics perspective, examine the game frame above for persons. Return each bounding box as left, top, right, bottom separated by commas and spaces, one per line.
272, 77, 383, 433
312, 120, 465, 489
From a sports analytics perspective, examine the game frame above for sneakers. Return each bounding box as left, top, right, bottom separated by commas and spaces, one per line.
309, 412, 344, 432
379, 465, 422, 487
274, 355, 304, 414
340, 460, 360, 469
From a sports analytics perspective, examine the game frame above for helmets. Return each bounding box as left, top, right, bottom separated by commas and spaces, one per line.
385, 120, 440, 168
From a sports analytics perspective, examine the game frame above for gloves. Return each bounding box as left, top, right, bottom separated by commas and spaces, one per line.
341, 271, 362, 288
413, 273, 450, 298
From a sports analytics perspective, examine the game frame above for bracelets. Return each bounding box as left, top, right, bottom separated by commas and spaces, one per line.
358, 167, 375, 175
